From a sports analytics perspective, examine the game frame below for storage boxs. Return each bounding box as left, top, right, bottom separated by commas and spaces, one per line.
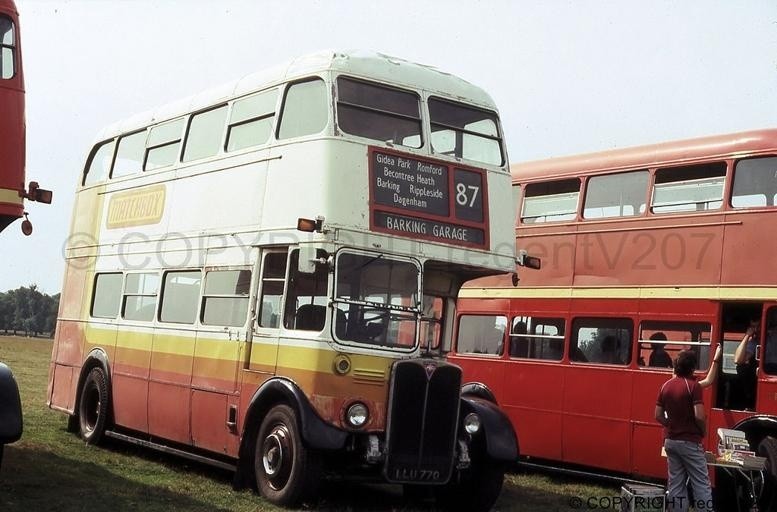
622, 487, 666, 512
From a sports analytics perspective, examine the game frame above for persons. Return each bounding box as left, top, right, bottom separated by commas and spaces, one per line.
541, 333, 563, 359
654, 349, 713, 511
662, 342, 722, 418
648, 332, 673, 368
509, 321, 530, 357
733, 314, 776, 412
594, 335, 627, 364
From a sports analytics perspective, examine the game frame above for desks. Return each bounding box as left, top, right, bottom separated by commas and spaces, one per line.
686, 453, 767, 511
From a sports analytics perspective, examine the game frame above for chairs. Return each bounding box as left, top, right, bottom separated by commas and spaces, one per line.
295, 304, 347, 340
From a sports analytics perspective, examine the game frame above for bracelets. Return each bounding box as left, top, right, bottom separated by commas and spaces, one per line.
711, 359, 719, 364
